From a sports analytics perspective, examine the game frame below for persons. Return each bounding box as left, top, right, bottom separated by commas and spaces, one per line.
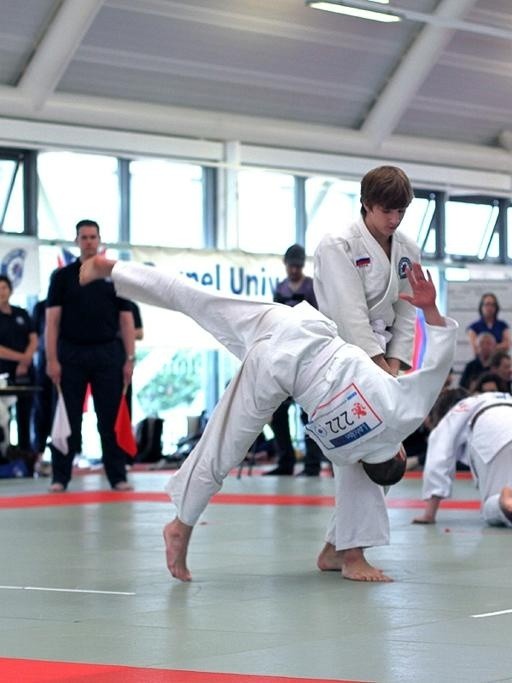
459, 291, 511, 398
258, 241, 323, 477
78, 254, 460, 584
1, 219, 142, 492
311, 165, 420, 583
414, 388, 512, 528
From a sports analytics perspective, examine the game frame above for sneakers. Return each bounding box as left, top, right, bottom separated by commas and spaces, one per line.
297, 467, 320, 475
267, 467, 294, 476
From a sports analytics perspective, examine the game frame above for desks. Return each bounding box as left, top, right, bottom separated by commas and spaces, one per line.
0, 386, 49, 477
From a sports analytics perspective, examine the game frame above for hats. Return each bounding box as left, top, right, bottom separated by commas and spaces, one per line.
287, 245, 305, 265
